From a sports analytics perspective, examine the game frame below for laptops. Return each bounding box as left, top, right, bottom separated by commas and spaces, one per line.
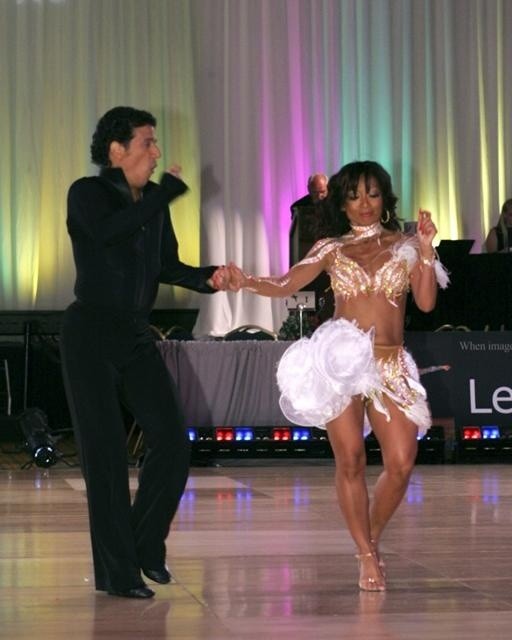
438, 240, 476, 254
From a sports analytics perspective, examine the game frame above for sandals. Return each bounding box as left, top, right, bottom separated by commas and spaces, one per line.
355, 539, 386, 591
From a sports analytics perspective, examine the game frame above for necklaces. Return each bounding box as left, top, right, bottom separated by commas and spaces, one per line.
339, 220, 384, 242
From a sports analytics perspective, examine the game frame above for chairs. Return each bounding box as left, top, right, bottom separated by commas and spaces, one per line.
126, 324, 280, 458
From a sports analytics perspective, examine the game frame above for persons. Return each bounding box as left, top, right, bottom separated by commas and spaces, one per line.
288, 170, 339, 316
481, 198, 512, 254
58, 105, 236, 596
215, 159, 439, 594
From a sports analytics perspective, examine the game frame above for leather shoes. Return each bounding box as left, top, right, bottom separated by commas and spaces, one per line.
142, 568, 171, 584
108, 587, 154, 598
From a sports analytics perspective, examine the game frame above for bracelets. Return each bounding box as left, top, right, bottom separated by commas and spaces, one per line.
419, 252, 437, 269
